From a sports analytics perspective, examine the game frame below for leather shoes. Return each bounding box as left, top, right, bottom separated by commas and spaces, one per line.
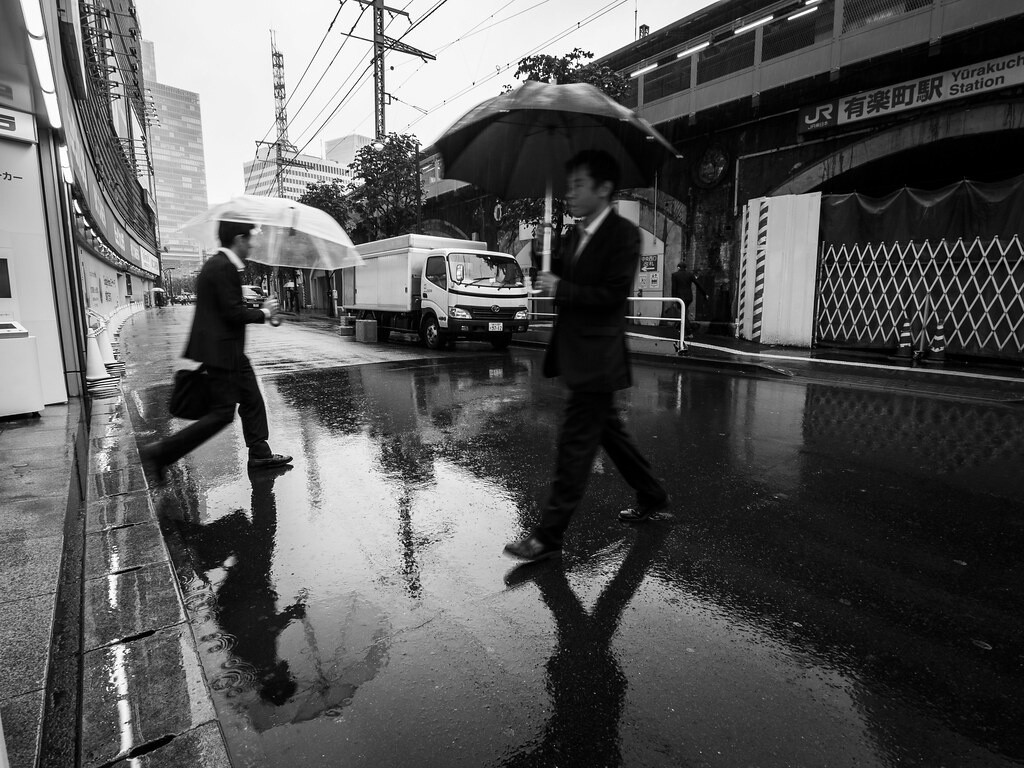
248, 455, 293, 470
619, 494, 669, 521
506, 536, 564, 558
143, 446, 166, 485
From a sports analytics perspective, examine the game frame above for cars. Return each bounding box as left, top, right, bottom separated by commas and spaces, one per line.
174, 292, 197, 304
241, 284, 269, 311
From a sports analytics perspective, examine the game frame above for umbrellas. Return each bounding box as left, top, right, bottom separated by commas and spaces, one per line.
176, 195, 366, 294
434, 79, 680, 273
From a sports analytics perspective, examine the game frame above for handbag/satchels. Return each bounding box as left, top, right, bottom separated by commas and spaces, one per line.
169, 362, 216, 420
662, 307, 680, 322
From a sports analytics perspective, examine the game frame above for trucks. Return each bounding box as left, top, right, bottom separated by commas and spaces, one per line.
334, 232, 531, 352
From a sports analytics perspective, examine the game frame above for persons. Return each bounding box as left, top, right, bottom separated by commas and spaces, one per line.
506, 148, 671, 559
139, 213, 293, 486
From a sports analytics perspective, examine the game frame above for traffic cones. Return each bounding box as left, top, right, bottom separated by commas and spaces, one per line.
888, 316, 913, 367
97, 322, 120, 365
84, 327, 111, 382
919, 318, 947, 368
